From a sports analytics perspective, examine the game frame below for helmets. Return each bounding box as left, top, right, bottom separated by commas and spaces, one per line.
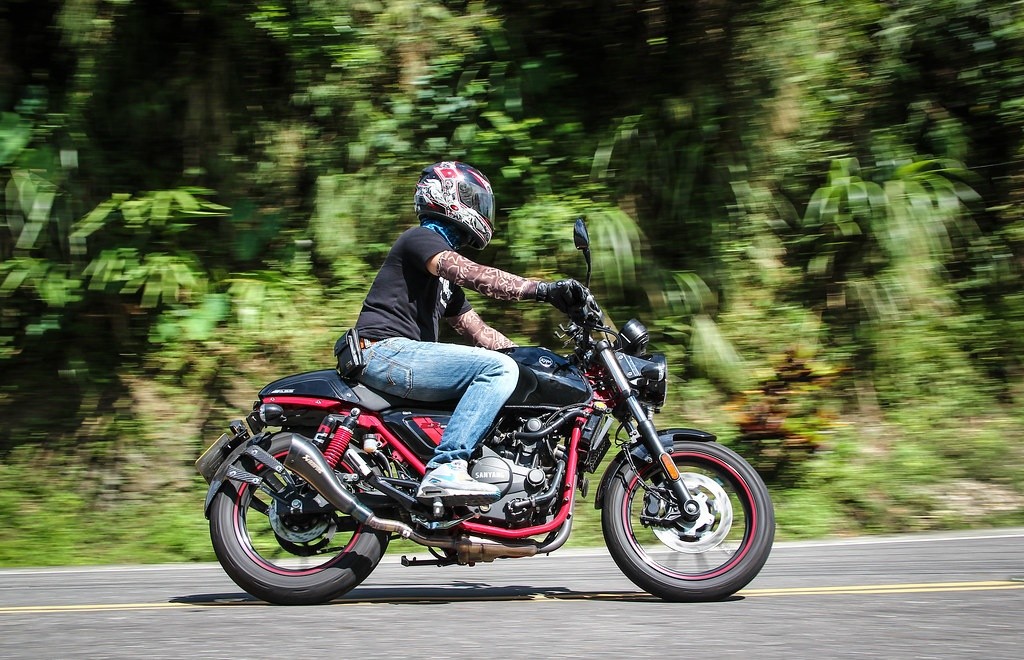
413, 160, 496, 250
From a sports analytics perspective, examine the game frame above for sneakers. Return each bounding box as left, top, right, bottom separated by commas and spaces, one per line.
416, 459, 501, 508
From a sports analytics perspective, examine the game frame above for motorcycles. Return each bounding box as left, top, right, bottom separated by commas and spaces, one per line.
194, 217, 778, 604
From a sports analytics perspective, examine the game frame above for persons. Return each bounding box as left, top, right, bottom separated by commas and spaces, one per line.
336, 161, 592, 500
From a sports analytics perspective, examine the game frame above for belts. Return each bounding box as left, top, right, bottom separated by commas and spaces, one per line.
360, 338, 376, 348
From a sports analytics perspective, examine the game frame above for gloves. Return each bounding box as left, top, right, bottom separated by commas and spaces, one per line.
536, 278, 587, 315
